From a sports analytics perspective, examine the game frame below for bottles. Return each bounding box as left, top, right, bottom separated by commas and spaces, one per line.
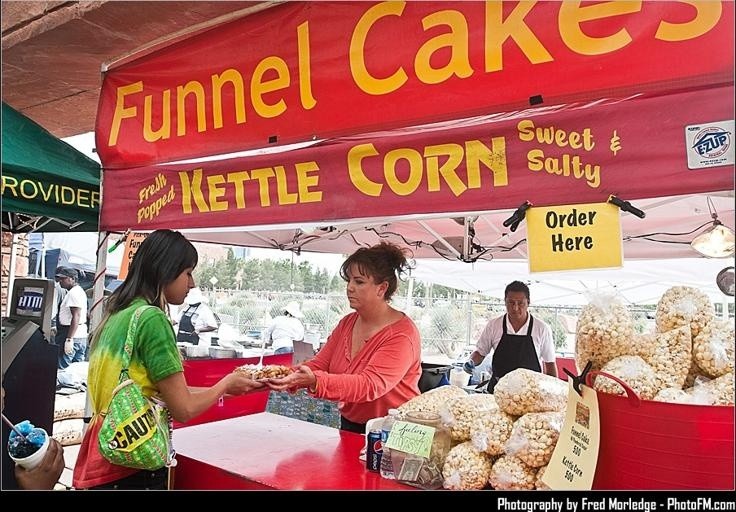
390, 412, 451, 489
440, 362, 471, 390
268, 386, 343, 430
380, 408, 400, 480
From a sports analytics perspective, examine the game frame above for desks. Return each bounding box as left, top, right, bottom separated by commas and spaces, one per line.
170, 412, 433, 490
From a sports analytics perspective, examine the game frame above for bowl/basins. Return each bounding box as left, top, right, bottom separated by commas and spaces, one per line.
207, 347, 236, 358
186, 345, 208, 356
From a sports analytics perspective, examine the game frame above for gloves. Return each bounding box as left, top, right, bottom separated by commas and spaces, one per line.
462, 359, 476, 375
64, 336, 74, 356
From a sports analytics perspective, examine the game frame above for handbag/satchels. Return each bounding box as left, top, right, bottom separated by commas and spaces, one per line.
95, 370, 177, 472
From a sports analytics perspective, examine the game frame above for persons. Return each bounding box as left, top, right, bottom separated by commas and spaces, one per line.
264, 241, 424, 434
51, 265, 90, 370
71, 227, 266, 491
2, 388, 66, 491
169, 288, 218, 347
261, 301, 305, 355
462, 280, 559, 394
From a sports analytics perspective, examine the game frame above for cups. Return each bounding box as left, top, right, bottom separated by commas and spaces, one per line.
8, 418, 49, 468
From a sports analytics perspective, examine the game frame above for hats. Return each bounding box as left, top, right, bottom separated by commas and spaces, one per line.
53, 268, 79, 281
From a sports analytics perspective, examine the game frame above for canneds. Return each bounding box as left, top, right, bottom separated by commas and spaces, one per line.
367, 430, 391, 472
